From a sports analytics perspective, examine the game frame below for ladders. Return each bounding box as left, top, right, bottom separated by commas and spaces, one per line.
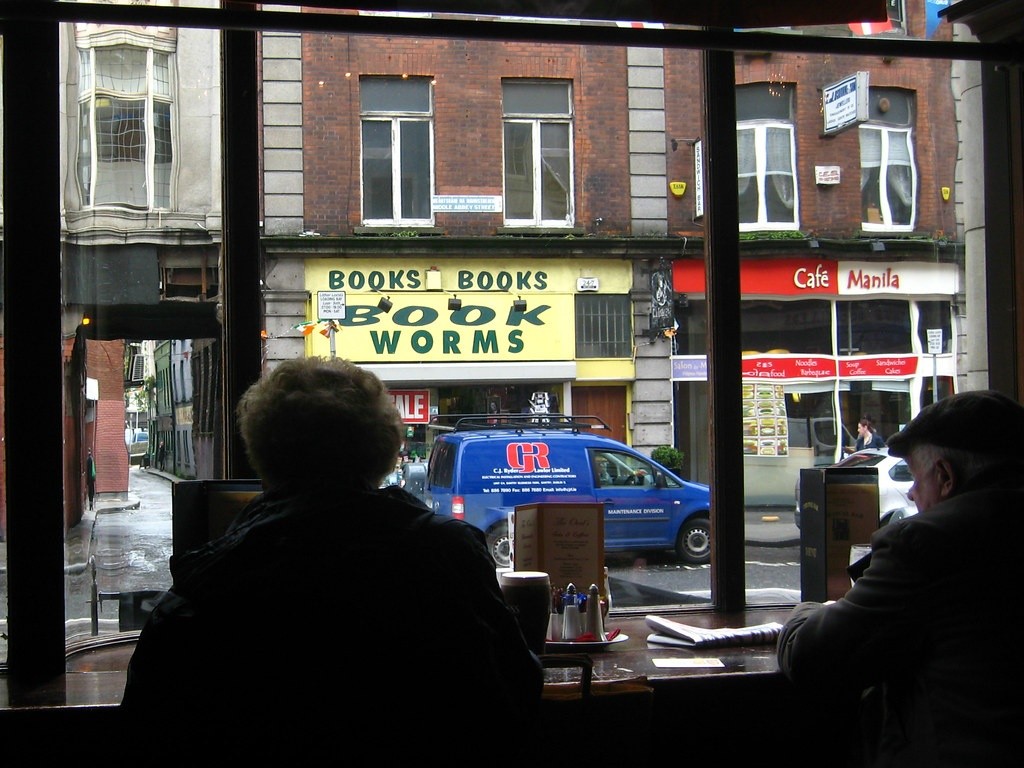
427, 412, 612, 431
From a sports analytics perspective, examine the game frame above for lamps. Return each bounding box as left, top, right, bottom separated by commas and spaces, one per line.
507, 290, 527, 312
442, 289, 462, 311
372, 286, 394, 313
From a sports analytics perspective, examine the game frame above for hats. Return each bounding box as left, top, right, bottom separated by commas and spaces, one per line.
886, 390, 1024, 458
595, 456, 607, 462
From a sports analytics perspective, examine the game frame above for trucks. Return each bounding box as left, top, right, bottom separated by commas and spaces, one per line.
742, 415, 862, 508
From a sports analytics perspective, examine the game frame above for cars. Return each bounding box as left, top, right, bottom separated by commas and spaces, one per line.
124, 427, 149, 457
794, 448, 919, 528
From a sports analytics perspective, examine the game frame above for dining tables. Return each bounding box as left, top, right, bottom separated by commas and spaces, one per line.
1, 600, 800, 714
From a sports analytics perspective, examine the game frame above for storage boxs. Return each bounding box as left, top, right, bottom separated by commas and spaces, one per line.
171, 479, 262, 556
798, 466, 882, 603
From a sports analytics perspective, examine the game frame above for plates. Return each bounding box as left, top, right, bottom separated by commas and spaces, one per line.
546, 632, 630, 649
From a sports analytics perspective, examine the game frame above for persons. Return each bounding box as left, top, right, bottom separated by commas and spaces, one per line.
85, 447, 96, 511
854, 418, 886, 451
115, 353, 545, 768
594, 455, 649, 486
773, 391, 1024, 768
158, 441, 165, 471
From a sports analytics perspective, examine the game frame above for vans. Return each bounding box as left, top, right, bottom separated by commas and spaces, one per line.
422, 415, 711, 567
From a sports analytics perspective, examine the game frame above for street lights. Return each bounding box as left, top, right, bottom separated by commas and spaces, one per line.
135, 390, 140, 429
